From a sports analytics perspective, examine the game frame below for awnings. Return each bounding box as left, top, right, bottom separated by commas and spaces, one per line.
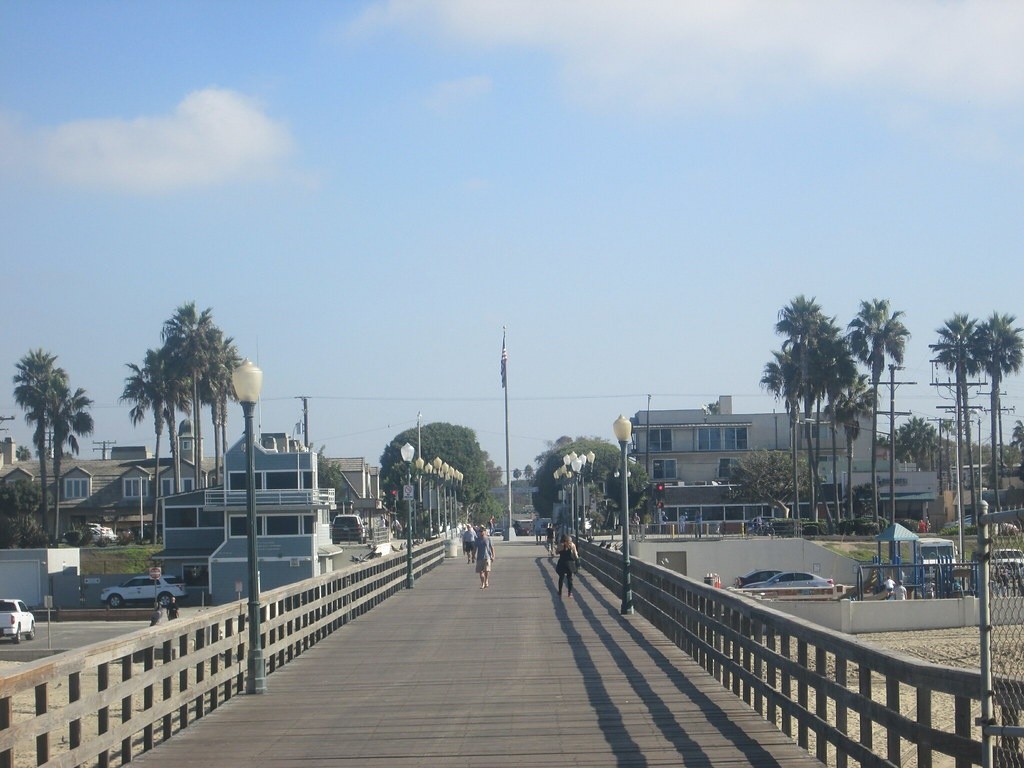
859, 492, 936, 501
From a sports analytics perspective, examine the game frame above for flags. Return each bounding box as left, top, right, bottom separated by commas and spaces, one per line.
501, 338, 508, 388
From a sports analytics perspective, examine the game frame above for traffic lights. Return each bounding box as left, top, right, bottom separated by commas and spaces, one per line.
656, 482, 664, 494
657, 500, 664, 509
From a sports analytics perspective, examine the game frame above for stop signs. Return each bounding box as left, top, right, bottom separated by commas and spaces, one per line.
150, 567, 160, 578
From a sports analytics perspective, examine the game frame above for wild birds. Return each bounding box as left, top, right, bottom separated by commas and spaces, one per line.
598, 540, 606, 548
399, 537, 425, 550
587, 535, 594, 543
350, 553, 367, 565
605, 542, 612, 549
390, 545, 401, 553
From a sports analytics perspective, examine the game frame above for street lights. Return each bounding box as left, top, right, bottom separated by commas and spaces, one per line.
613, 413, 634, 614
415, 457, 425, 544
458, 473, 463, 500
972, 408, 983, 499
447, 466, 454, 539
555, 452, 595, 557
229, 355, 269, 696
803, 418, 895, 562
434, 456, 442, 539
424, 462, 433, 541
454, 468, 459, 537
441, 461, 449, 537
400, 442, 415, 589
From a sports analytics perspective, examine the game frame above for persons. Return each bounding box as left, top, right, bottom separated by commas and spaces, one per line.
632, 512, 640, 533
755, 513, 763, 536
918, 520, 926, 533
534, 519, 542, 545
167, 597, 180, 621
376, 516, 385, 528
884, 577, 896, 597
556, 535, 578, 597
489, 516, 495, 535
695, 512, 702, 538
680, 513, 686, 534
392, 518, 402, 537
462, 524, 494, 589
1019, 563, 1024, 595
895, 581, 907, 600
545, 523, 555, 555
662, 512, 668, 524
1001, 569, 1010, 597
955, 580, 960, 590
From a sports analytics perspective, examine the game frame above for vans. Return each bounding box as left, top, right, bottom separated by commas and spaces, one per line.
907, 537, 959, 581
491, 517, 553, 538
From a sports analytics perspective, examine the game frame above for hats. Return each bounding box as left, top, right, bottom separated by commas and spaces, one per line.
479, 527, 486, 532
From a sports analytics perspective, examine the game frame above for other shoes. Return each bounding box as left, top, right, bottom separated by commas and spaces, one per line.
568, 592, 572, 597
480, 586, 485, 589
485, 582, 488, 587
557, 592, 561, 596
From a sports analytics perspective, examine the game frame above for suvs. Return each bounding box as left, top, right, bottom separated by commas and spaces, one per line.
330, 513, 368, 545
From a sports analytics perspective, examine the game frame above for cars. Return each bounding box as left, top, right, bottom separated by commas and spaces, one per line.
742, 571, 834, 596
988, 548, 1024, 583
84, 522, 120, 548
100, 574, 188, 609
736, 569, 783, 589
0, 599, 36, 644
